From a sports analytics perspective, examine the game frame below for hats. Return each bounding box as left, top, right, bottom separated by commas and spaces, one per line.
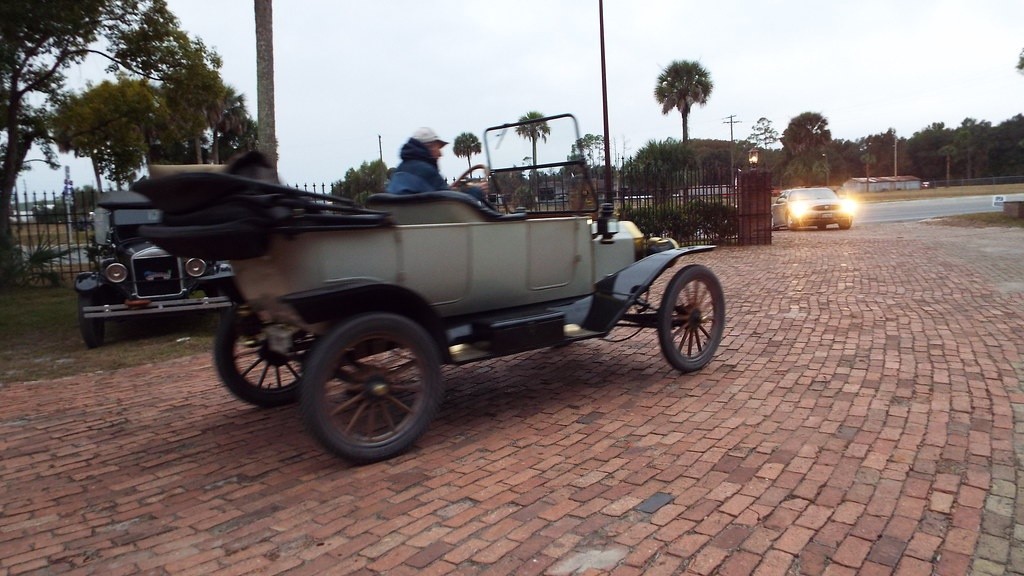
411, 126, 449, 148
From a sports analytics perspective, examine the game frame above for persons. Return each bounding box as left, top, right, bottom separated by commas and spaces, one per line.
384, 126, 489, 205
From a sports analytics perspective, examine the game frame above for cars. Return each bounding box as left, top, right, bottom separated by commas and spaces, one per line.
74, 190, 238, 349
771, 188, 853, 231
128, 113, 725, 465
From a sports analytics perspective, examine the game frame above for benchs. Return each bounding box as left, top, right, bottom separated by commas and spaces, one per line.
365, 191, 527, 224
